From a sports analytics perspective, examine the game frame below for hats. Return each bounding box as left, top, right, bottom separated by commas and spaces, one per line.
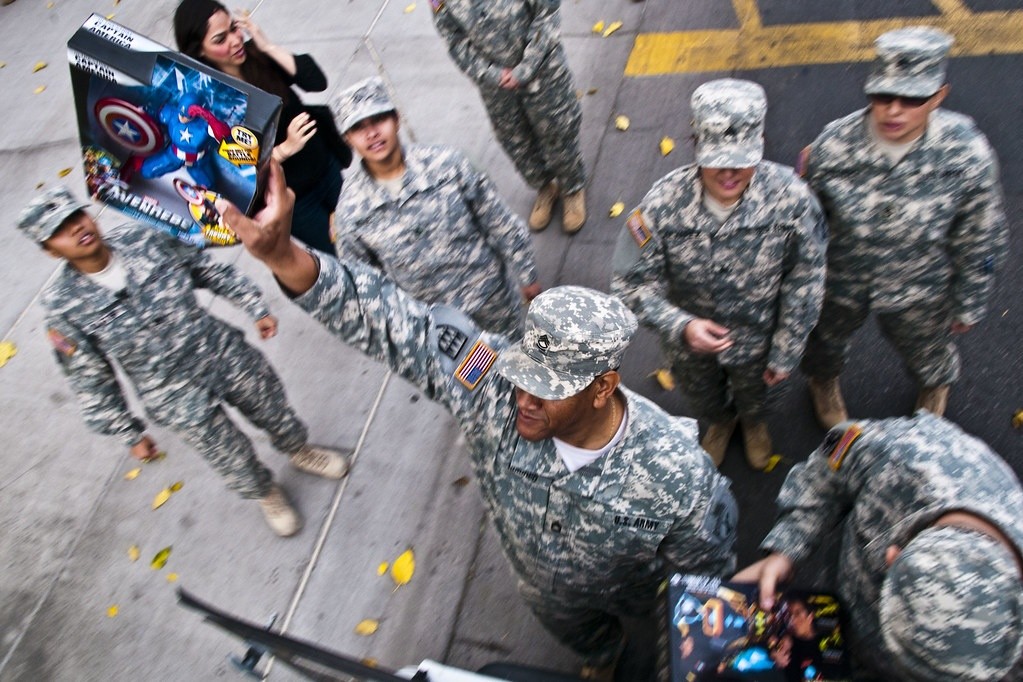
866, 24, 956, 98
880, 526, 1023, 682
497, 285, 640, 399
693, 78, 767, 169
15, 184, 93, 242
329, 76, 395, 134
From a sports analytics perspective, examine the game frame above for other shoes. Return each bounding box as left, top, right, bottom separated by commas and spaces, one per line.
912, 387, 948, 418
743, 419, 772, 467
701, 414, 737, 466
530, 181, 559, 229
291, 447, 349, 479
561, 187, 586, 232
579, 633, 625, 682
810, 378, 848, 429
262, 485, 300, 537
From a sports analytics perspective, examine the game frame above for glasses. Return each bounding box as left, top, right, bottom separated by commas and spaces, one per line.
868, 93, 939, 107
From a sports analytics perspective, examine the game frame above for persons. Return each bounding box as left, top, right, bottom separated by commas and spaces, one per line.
613, 76, 828, 469
771, 599, 828, 681
796, 27, 1010, 422
135, 91, 230, 187
734, 407, 1021, 682
197, 185, 223, 228
215, 156, 739, 681
325, 76, 545, 344
172, 0, 353, 256
431, 0, 588, 234
15, 183, 349, 538
